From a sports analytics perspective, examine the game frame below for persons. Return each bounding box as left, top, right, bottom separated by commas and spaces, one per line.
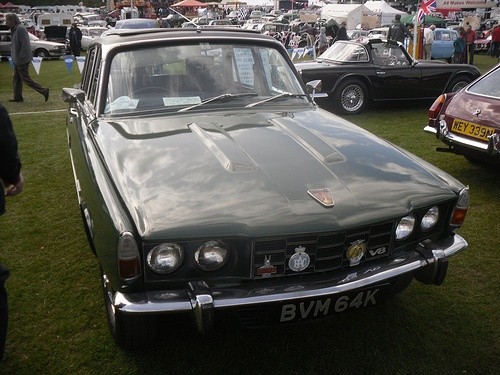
487, 23, 500, 59
78, 0, 360, 59
453, 29, 466, 64
0, 101, 23, 287
422, 24, 436, 60
69, 23, 82, 57
387, 14, 413, 46
4, 12, 49, 102
465, 24, 476, 65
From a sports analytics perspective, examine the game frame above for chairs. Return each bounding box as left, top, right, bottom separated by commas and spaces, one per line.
114, 56, 225, 95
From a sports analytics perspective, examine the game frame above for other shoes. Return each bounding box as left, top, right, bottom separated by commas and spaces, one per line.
44, 88, 49, 102
9, 98, 23, 102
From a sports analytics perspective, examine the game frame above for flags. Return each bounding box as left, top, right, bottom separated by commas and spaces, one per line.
417, 0, 437, 22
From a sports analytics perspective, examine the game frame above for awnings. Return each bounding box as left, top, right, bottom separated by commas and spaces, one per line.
0, 2, 20, 13
171, 0, 273, 17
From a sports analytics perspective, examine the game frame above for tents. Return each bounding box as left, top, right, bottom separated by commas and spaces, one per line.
364, 1, 408, 27
321, 3, 381, 36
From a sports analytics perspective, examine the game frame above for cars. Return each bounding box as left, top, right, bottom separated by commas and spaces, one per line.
424, 62, 500, 165
61, 29, 470, 356
0, 31, 66, 59
430, 28, 460, 58
0, 4, 500, 59
295, 40, 483, 115
